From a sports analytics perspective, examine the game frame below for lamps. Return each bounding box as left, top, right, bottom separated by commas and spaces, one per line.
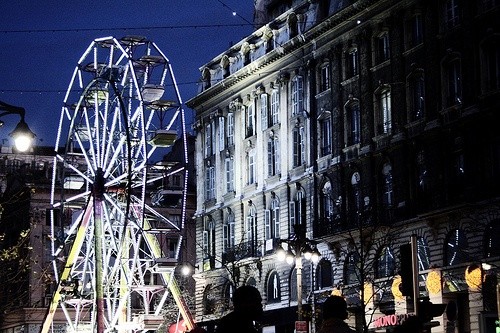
180, 260, 199, 276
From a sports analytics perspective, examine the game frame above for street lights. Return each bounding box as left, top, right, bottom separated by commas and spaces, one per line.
273, 224, 322, 321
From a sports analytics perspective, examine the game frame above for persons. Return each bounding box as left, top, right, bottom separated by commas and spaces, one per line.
316, 296, 356, 333
216, 285, 263, 333
399, 301, 435, 333
